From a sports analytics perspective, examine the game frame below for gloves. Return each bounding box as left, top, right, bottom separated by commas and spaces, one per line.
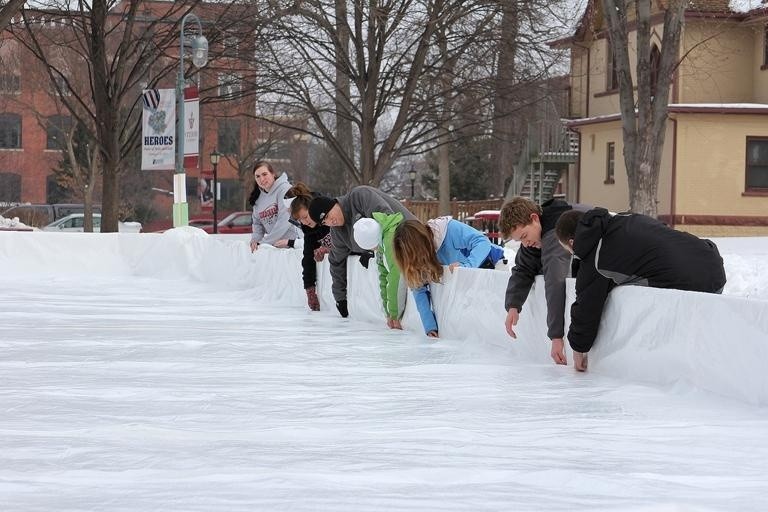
359, 251, 374, 270
335, 300, 348, 318
314, 246, 330, 262
306, 286, 320, 312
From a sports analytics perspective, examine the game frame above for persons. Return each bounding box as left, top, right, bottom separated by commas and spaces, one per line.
286, 196, 332, 311
392, 215, 504, 338
249, 161, 297, 253
308, 185, 418, 318
275, 183, 310, 248
499, 196, 594, 365
353, 212, 407, 329
556, 207, 727, 372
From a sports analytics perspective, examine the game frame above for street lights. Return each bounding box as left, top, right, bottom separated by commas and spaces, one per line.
0, 201, 32, 216
172, 11, 212, 227
210, 146, 219, 233
408, 164, 418, 198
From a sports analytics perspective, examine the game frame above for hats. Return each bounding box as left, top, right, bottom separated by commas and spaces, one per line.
353, 217, 382, 250
308, 196, 337, 223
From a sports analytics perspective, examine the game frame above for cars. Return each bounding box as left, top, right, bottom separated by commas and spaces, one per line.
40, 204, 144, 233
153, 219, 222, 233
202, 212, 253, 235
